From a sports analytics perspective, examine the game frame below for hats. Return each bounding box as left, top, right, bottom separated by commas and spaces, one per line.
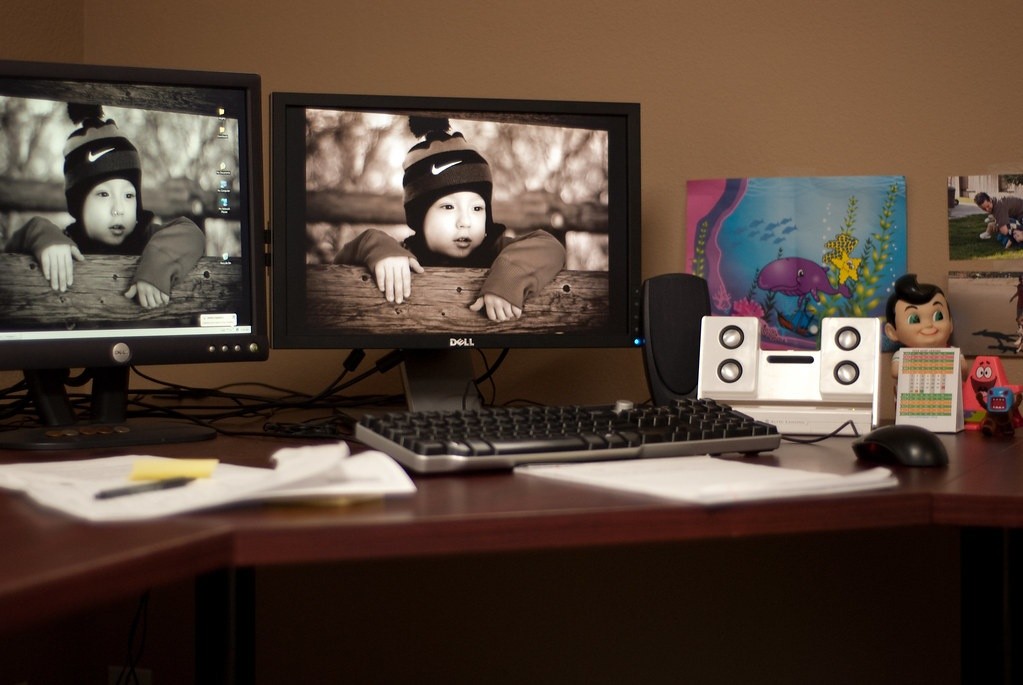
62, 101, 144, 220
403, 115, 493, 239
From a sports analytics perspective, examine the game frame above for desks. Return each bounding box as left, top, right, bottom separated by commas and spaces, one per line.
0, 396, 1023, 685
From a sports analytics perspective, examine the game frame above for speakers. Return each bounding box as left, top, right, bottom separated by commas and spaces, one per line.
644, 273, 897, 437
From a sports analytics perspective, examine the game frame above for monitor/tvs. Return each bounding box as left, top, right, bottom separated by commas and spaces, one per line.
269, 92, 643, 412
0, 60, 268, 452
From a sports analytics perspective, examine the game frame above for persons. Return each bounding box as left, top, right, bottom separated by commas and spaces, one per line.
331, 116, 565, 321
884, 273, 969, 406
4, 101, 206, 307
974, 192, 1023, 248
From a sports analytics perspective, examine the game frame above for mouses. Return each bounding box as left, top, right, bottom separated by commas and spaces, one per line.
850, 425, 949, 470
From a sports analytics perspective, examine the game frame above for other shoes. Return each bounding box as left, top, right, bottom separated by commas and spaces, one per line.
984, 214, 993, 223
980, 232, 991, 239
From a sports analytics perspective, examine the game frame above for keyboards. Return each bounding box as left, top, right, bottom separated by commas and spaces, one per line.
354, 398, 781, 474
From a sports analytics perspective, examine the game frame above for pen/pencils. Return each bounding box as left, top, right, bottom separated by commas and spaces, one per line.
96, 475, 196, 500
